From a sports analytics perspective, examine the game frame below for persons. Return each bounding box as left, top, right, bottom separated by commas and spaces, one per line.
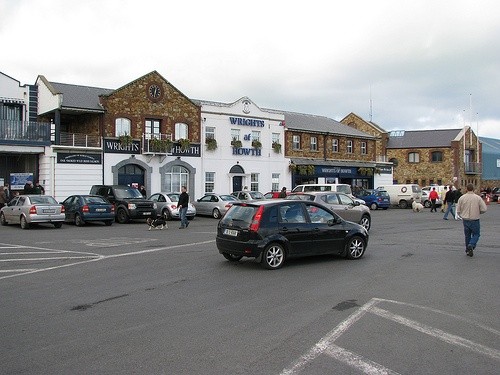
454, 189, 463, 221
0, 184, 19, 210
429, 188, 439, 213
33, 180, 44, 194
24, 181, 36, 195
457, 184, 487, 257
443, 185, 455, 220
440, 187, 447, 212
177, 186, 189, 229
278, 187, 287, 198
244, 186, 248, 191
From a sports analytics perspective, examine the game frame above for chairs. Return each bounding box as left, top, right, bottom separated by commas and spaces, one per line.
162, 196, 166, 202
285, 207, 299, 218
173, 196, 177, 202
328, 196, 336, 201
211, 196, 217, 202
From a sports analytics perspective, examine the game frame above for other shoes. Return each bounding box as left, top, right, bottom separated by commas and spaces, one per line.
186, 222, 189, 228
454, 217, 462, 221
430, 208, 445, 213
442, 218, 448, 221
179, 225, 185, 229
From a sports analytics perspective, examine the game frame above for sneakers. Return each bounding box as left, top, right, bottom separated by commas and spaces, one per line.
468, 244, 474, 257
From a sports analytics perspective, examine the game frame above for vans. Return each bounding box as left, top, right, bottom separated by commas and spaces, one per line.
373, 184, 421, 210
287, 183, 352, 200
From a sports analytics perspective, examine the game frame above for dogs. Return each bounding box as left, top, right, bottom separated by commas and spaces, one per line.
412, 201, 424, 212
146, 212, 169, 230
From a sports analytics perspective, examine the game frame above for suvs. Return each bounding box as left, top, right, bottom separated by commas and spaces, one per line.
89, 185, 158, 224
281, 191, 371, 232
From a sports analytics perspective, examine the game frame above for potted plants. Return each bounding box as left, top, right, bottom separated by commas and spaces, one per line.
150, 135, 173, 154
205, 136, 218, 151
232, 137, 243, 150
358, 167, 383, 177
272, 140, 282, 154
252, 138, 263, 151
308, 164, 315, 176
119, 130, 134, 149
178, 138, 191, 153
298, 164, 308, 176
289, 159, 297, 175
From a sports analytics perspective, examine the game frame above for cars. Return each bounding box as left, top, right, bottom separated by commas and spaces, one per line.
148, 192, 196, 221
264, 190, 291, 199
353, 189, 391, 211
193, 194, 241, 220
0, 194, 66, 230
421, 186, 457, 208
491, 187, 500, 202
59, 194, 116, 227
226, 191, 266, 201
215, 199, 370, 271
